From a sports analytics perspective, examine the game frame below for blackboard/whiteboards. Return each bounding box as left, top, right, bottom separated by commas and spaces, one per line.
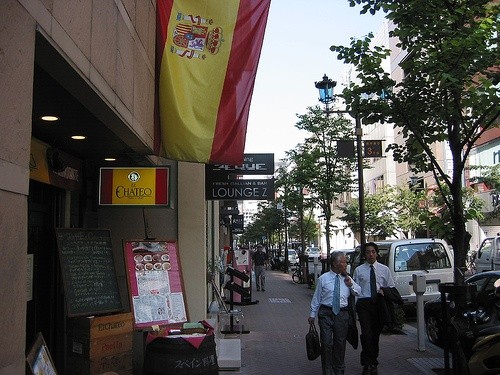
55, 227, 123, 317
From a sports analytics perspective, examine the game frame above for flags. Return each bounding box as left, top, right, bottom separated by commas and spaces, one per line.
154, 0, 271, 166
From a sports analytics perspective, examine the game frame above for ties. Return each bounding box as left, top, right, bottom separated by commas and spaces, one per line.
332, 275, 341, 315
370, 265, 378, 304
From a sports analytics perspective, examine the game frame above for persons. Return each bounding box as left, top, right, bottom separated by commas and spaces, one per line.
308, 251, 361, 375
251, 245, 268, 292
353, 242, 404, 375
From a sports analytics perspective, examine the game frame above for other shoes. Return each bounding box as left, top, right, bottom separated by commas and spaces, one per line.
362, 363, 377, 375
262, 288, 265, 291
257, 288, 260, 291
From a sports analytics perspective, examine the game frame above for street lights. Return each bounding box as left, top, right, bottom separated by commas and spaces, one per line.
315, 73, 394, 265
409, 171, 443, 239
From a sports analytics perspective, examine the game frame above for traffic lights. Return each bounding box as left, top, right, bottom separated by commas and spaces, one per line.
418, 199, 426, 209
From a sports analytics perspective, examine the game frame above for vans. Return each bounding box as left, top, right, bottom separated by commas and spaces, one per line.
349, 237, 454, 321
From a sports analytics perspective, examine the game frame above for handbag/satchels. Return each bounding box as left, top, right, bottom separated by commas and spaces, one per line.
305, 321, 320, 360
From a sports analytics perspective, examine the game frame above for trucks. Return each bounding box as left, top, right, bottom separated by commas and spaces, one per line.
473, 231, 500, 296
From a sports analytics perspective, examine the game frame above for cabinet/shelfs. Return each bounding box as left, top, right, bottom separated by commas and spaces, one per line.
69, 313, 134, 375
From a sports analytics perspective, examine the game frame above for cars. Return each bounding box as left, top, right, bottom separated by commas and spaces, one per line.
331, 248, 356, 278
424, 270, 500, 349
268, 247, 327, 265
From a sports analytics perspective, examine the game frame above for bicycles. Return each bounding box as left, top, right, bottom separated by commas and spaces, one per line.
292, 263, 302, 284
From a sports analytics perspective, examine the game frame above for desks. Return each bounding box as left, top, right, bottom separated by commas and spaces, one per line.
217, 310, 242, 349
